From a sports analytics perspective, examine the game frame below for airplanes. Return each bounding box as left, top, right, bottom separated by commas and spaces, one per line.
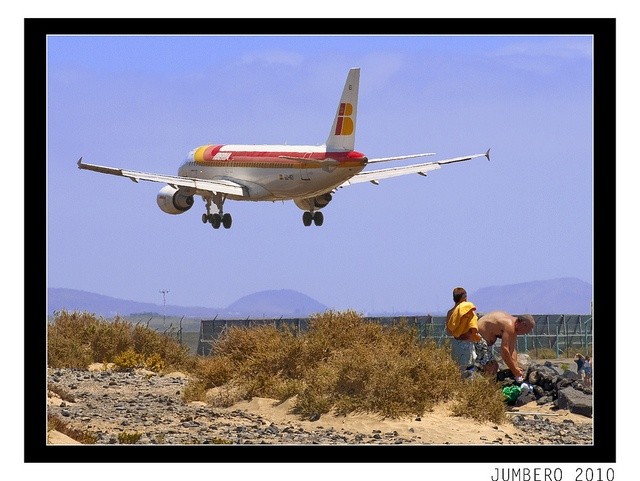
76, 68, 493, 229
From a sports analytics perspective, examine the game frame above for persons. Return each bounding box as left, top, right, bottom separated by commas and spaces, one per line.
574, 353, 586, 378
582, 355, 592, 387
446, 287, 482, 384
473, 312, 536, 379
